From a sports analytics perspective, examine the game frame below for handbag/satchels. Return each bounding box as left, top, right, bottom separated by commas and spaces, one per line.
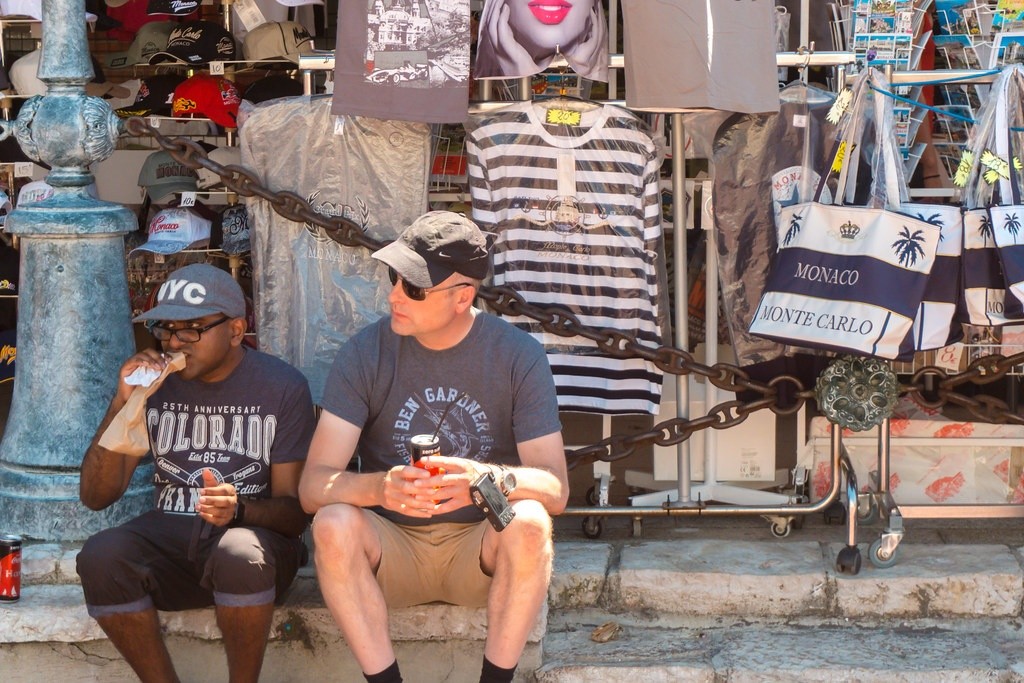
746, 59, 1024, 363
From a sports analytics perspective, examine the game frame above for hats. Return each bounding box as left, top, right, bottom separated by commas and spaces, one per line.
132, 262, 248, 322
0, 328, 17, 381
370, 211, 490, 288
0, 245, 20, 298
0, 0, 335, 254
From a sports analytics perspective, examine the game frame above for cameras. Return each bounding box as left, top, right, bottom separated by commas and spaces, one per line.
469, 472, 516, 532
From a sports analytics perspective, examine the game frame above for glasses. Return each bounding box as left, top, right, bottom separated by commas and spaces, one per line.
148, 316, 231, 343
388, 267, 473, 302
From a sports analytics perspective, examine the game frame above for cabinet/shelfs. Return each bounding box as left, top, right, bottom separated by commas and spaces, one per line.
301, 0, 617, 312
135, 0, 316, 285
0, 20, 43, 384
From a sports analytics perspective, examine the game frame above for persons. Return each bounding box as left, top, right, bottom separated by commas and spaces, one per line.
298, 210, 571, 683
76, 264, 316, 683
476, 0, 608, 76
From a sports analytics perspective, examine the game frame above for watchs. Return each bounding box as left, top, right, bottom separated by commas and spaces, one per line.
228, 498, 246, 527
495, 464, 517, 499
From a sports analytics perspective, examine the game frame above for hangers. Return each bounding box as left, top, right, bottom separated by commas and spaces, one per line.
531, 63, 604, 108
779, 51, 837, 108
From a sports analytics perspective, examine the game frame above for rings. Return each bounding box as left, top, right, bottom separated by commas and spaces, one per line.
400, 505, 406, 512
